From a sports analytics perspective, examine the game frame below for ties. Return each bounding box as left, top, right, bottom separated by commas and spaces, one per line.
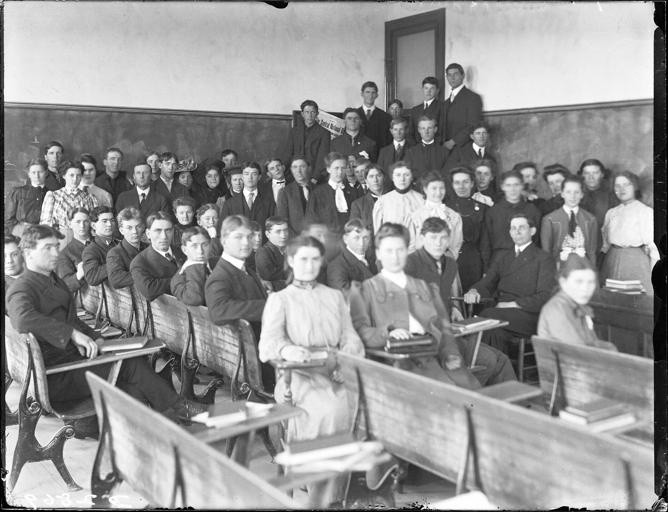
568, 209, 578, 238
396, 143, 402, 159
85, 239, 91, 247
365, 108, 373, 121
248, 192, 255, 211
164, 252, 179, 268
515, 247, 521, 258
436, 260, 444, 276
478, 148, 483, 163
83, 185, 90, 195
425, 102, 429, 111
140, 193, 146, 209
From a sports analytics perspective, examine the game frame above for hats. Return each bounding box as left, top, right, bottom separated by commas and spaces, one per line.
174, 158, 199, 174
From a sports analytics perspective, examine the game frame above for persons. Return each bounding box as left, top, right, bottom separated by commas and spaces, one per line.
407, 76, 447, 144
285, 100, 330, 184
443, 121, 497, 169
332, 107, 378, 163
403, 115, 449, 175
388, 98, 403, 118
445, 63, 483, 150
355, 82, 393, 146
376, 117, 412, 174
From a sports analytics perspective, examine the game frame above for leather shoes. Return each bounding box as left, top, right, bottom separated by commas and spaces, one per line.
172, 399, 213, 425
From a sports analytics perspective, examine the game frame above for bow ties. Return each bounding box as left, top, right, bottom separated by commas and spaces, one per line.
574, 304, 597, 319
426, 202, 448, 220
276, 181, 286, 184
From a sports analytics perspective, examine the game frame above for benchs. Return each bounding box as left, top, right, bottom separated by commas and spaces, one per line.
3, 280, 658, 511
